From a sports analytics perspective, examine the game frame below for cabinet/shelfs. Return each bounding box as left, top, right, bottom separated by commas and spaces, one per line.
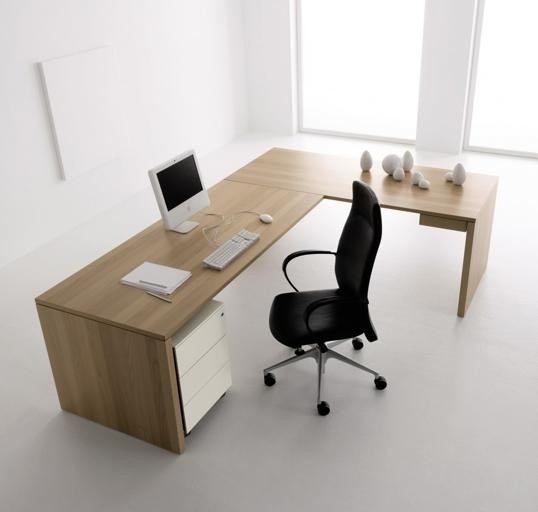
171, 300, 232, 436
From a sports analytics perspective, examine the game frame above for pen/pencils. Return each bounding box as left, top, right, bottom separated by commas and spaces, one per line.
145, 291, 172, 303
139, 280, 166, 288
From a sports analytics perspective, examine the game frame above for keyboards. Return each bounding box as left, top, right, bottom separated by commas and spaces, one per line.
202, 229, 259, 270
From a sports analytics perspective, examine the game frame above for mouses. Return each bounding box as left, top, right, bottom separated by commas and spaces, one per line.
260, 214, 273, 223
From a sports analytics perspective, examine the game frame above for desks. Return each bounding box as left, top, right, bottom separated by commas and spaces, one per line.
35, 179, 325, 454
223, 148, 499, 318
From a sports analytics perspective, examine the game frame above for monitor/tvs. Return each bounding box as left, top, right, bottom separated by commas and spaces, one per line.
148, 149, 210, 234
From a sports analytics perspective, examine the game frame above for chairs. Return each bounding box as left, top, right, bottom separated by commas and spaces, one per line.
264, 180, 389, 416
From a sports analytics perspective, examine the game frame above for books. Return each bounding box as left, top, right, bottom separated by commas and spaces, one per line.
120, 261, 192, 296
